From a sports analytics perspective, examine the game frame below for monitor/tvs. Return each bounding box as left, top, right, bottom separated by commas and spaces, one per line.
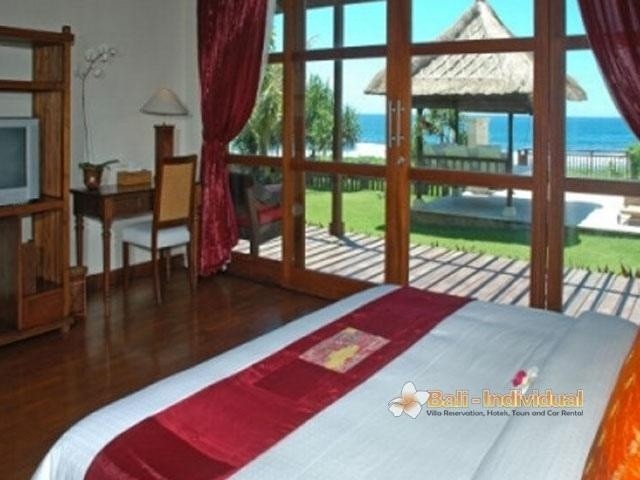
0, 117, 40, 206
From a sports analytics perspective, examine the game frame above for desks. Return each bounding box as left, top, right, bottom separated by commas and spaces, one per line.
68, 181, 204, 301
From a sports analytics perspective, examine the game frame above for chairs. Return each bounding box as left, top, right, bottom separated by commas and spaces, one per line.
229, 171, 284, 256
123, 155, 197, 303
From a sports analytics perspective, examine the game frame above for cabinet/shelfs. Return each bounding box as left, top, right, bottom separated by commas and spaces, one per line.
0, 25, 88, 348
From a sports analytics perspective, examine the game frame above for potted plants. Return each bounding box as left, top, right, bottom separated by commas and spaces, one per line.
75, 42, 120, 194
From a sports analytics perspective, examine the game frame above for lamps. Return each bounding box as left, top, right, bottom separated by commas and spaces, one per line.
139, 87, 188, 184
